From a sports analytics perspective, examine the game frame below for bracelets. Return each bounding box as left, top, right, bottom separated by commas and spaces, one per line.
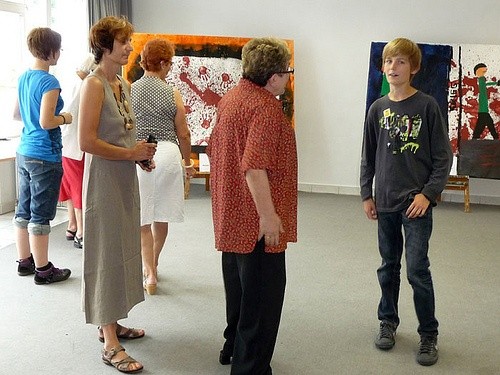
59, 114, 65, 125
182, 159, 194, 168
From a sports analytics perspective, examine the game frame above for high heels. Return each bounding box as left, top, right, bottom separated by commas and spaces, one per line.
142, 274, 158, 296
144, 269, 157, 279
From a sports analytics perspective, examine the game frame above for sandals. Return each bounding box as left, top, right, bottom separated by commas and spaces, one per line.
101, 346, 144, 374
96, 321, 145, 343
73, 236, 83, 249
65, 230, 77, 241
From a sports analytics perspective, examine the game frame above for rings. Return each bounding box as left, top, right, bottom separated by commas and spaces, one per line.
265, 235, 269, 238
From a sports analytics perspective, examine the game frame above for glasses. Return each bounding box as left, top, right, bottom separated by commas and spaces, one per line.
163, 60, 175, 66
271, 66, 295, 76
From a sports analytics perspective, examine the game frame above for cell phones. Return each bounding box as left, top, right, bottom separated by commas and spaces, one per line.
142, 139, 157, 168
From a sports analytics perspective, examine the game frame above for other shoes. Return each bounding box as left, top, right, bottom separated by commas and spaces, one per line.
218, 349, 233, 366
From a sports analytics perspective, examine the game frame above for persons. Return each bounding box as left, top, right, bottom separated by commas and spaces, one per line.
12, 27, 73, 285
129, 40, 193, 294
80, 16, 158, 374
59, 53, 96, 249
359, 38, 452, 366
207, 38, 297, 375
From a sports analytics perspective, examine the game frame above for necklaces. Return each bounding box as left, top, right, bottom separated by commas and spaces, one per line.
111, 75, 135, 130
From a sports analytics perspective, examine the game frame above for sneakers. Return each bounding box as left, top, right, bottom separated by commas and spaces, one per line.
33, 262, 71, 284
415, 330, 440, 366
374, 319, 399, 349
15, 253, 36, 276
